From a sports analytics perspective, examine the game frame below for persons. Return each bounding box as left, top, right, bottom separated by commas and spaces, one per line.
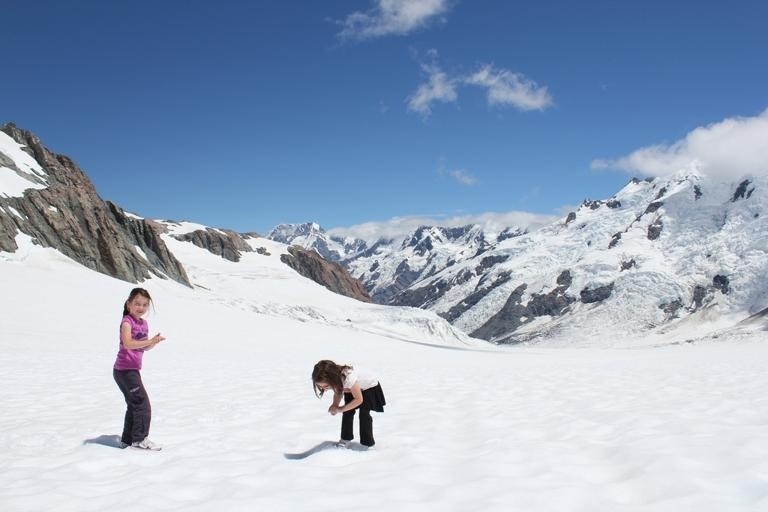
112, 287, 167, 452
312, 359, 388, 450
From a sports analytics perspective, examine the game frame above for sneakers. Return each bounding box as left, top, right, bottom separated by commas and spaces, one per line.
336, 438, 350, 448
119, 442, 131, 448
133, 436, 160, 450
